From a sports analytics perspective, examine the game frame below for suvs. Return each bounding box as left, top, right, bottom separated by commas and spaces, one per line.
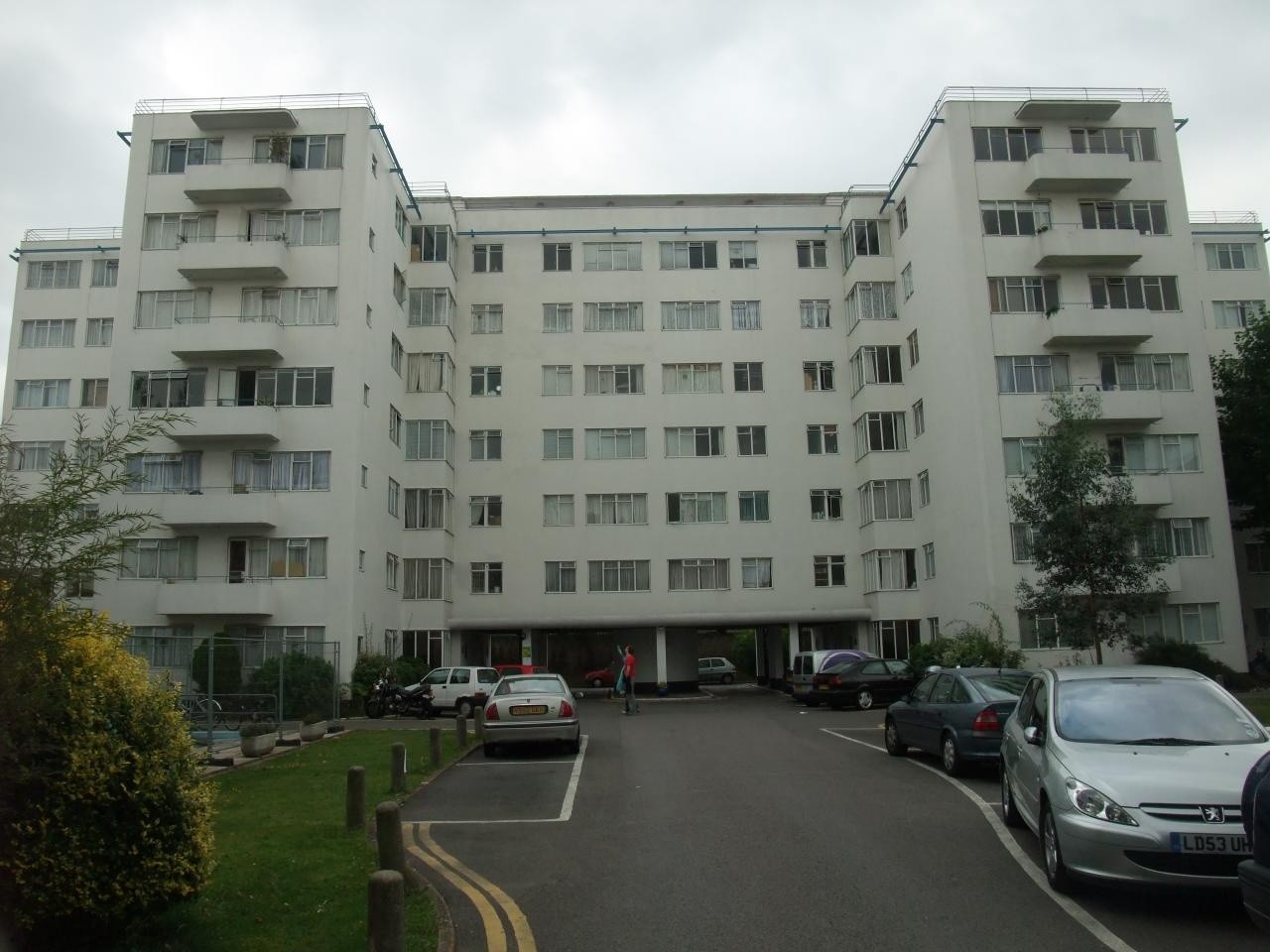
405, 666, 503, 718
490, 664, 549, 677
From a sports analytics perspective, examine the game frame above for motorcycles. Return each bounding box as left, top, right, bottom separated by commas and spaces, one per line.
364, 668, 436, 719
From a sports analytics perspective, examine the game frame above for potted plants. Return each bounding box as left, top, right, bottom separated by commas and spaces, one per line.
239, 722, 280, 758
299, 712, 331, 742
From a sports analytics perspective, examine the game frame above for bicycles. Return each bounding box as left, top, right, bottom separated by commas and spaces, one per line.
168, 682, 222, 731
222, 699, 279, 733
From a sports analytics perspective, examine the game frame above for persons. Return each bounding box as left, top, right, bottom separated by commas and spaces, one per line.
615, 643, 636, 714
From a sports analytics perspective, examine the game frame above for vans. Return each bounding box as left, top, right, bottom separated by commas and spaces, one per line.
793, 649, 877, 699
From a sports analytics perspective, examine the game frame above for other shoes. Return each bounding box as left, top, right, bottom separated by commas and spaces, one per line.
622, 710, 631, 714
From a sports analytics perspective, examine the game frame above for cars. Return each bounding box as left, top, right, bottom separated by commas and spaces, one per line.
996, 666, 1270, 899
481, 673, 585, 757
884, 666, 1039, 777
698, 657, 739, 685
809, 657, 925, 710
585, 660, 620, 686
